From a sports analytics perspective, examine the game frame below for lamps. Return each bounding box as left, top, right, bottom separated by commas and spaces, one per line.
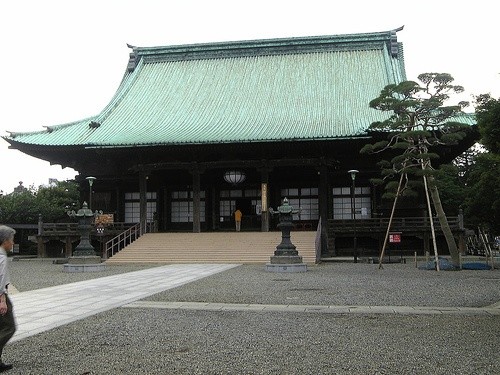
224, 169, 248, 184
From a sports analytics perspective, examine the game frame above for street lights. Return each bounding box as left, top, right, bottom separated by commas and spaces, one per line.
85, 176, 97, 244
347, 169, 359, 263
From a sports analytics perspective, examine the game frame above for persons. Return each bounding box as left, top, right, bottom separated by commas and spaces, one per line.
233, 209, 243, 231
0, 226, 17, 373
